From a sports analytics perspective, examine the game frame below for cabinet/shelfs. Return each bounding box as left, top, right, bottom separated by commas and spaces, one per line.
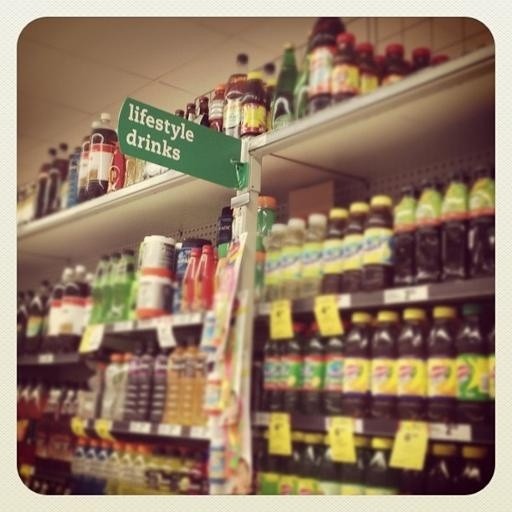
17, 45, 495, 495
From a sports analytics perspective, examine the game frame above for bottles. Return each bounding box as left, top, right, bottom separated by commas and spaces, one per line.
254, 165, 495, 304
82, 334, 210, 427
67, 436, 208, 494
259, 299, 490, 432
253, 426, 490, 495
32, 16, 431, 219
17, 247, 137, 355
182, 243, 229, 315
